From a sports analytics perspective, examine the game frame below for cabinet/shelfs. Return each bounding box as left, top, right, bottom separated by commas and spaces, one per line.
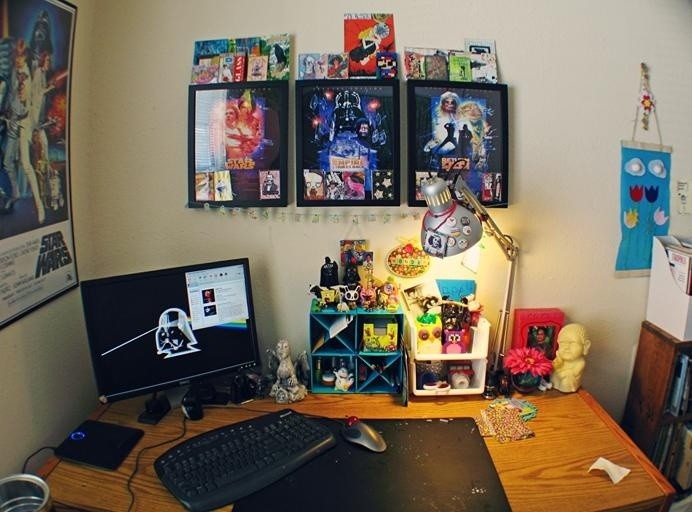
621, 320, 692, 501
308, 299, 405, 395
405, 312, 490, 396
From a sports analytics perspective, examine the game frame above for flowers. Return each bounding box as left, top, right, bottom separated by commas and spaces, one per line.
504, 347, 554, 379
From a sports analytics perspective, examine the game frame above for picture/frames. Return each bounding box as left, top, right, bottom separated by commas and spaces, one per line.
294, 77, 401, 207
406, 78, 509, 209
0, 0, 79, 331
512, 307, 564, 360
187, 79, 290, 209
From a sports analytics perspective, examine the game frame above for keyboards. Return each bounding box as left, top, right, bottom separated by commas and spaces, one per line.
152, 408, 334, 512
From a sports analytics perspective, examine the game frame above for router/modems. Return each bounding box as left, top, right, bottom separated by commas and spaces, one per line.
51, 418, 145, 471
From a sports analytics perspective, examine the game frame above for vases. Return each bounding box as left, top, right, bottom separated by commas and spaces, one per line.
510, 371, 542, 394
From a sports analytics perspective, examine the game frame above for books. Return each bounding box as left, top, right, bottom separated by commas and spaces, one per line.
664, 239, 692, 295
645, 350, 692, 496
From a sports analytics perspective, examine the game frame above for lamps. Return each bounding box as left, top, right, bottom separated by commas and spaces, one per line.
421, 159, 520, 399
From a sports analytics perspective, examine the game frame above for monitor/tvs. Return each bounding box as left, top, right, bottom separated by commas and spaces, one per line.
78, 256, 262, 422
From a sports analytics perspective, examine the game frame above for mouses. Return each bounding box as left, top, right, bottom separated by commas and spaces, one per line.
340, 416, 389, 455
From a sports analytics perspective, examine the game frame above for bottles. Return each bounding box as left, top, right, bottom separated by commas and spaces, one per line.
315, 360, 323, 384
0, 473, 54, 512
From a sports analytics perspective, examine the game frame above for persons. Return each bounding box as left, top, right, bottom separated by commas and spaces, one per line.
4, 38, 55, 223
430, 92, 490, 164
223, 97, 259, 157
531, 329, 554, 351
326, 180, 342, 199
548, 323, 591, 394
356, 119, 370, 141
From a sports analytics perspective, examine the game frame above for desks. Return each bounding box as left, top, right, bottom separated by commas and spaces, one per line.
35, 386, 676, 512
